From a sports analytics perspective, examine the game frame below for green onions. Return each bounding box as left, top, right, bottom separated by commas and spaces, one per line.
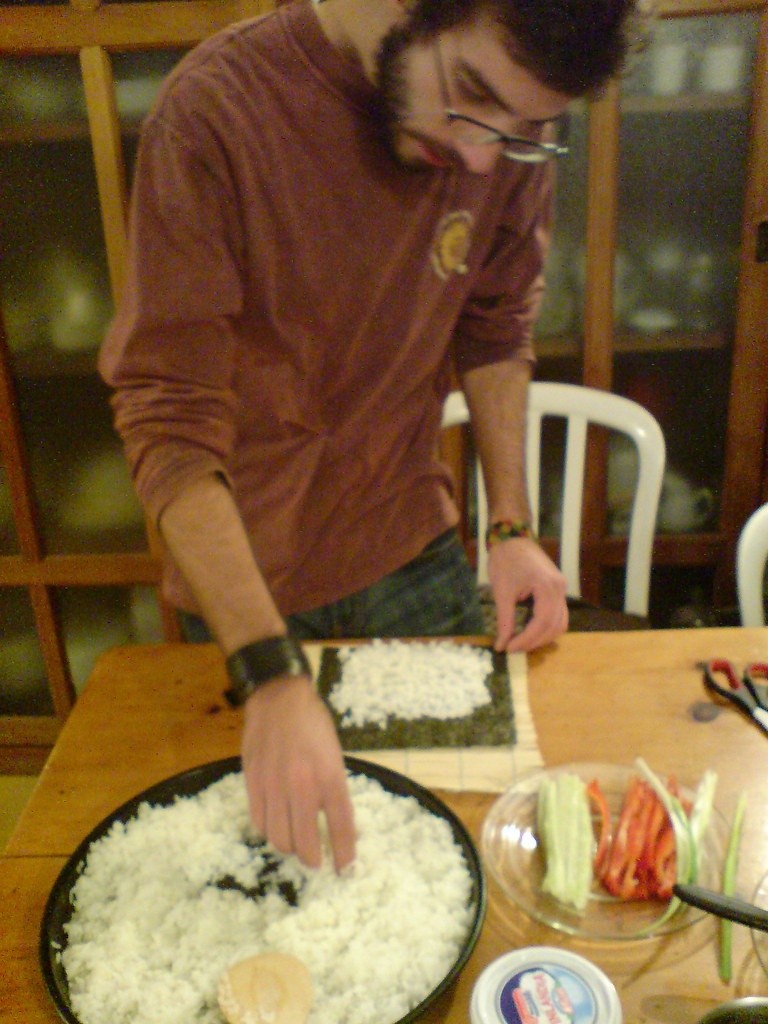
635, 756, 750, 983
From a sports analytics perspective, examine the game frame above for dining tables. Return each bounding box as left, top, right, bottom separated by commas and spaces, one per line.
1, 625, 768, 1024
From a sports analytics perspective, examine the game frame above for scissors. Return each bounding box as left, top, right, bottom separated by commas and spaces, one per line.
702, 656, 768, 739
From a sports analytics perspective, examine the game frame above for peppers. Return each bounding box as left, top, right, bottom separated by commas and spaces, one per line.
586, 772, 693, 904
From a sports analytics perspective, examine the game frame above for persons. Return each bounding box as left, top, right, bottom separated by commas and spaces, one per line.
109, 0, 640, 875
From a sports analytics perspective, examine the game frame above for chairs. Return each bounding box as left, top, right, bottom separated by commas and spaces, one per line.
442, 380, 666, 634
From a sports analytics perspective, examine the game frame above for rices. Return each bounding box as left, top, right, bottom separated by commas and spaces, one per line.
50, 758, 478, 1024
326, 635, 495, 730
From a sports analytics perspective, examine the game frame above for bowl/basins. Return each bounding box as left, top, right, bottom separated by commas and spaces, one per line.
697, 996, 768, 1024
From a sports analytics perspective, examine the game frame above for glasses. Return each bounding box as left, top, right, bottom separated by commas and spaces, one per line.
431, 23, 571, 163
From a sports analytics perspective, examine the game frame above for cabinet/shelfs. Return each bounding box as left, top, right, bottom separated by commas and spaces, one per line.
0, 0, 767, 779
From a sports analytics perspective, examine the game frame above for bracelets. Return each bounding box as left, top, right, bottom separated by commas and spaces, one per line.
486, 521, 536, 549
223, 636, 309, 703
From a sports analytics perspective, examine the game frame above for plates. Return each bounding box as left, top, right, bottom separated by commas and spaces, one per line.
39, 754, 487, 1024
749, 868, 768, 979
482, 762, 738, 941
